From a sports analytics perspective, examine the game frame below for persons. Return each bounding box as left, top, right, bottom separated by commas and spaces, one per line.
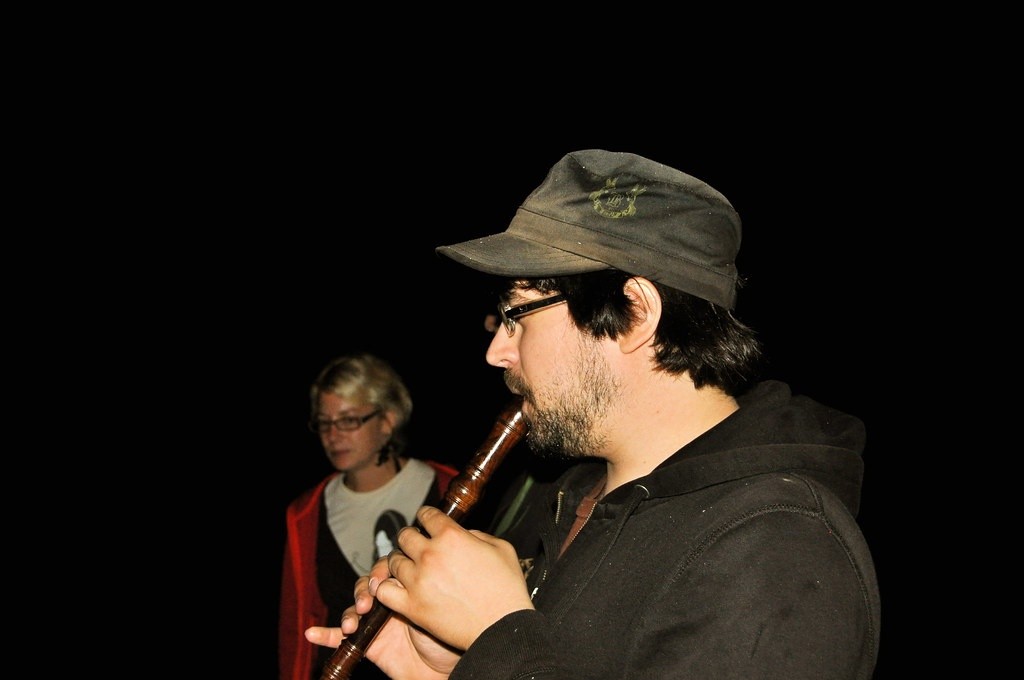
279, 357, 460, 680
306, 148, 880, 680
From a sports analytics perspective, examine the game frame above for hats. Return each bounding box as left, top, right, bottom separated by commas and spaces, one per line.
435, 146, 742, 306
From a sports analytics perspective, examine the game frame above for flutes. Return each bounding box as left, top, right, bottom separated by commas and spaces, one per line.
317, 395, 526, 679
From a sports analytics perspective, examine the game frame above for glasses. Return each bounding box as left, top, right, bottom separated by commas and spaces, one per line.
495, 291, 572, 336
307, 405, 385, 433
